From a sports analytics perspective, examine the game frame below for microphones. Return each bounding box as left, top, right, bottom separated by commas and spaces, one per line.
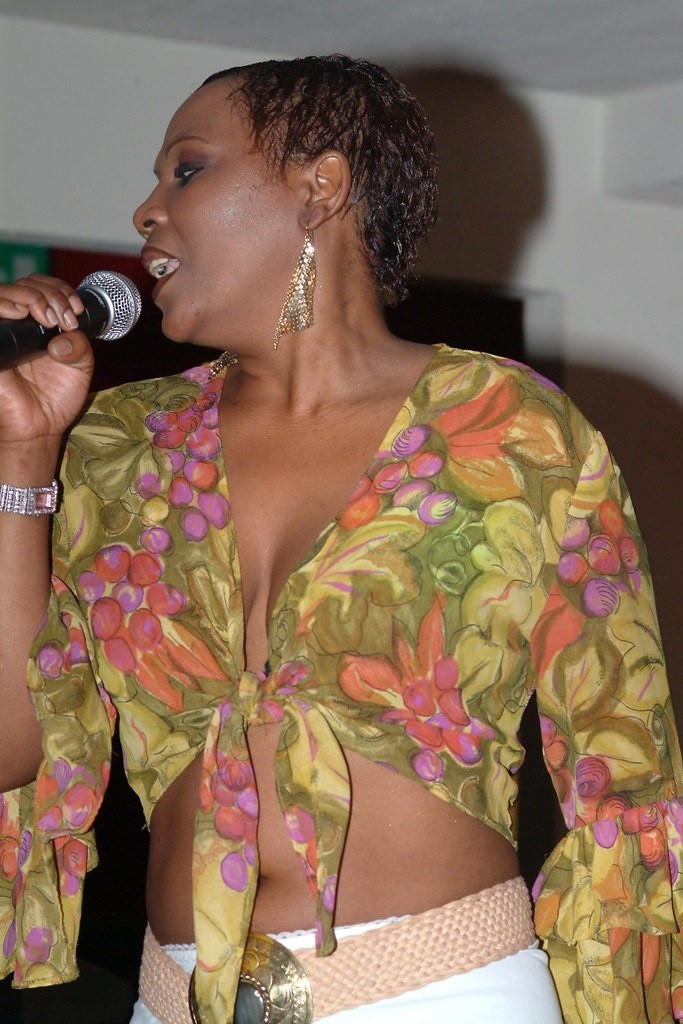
0, 271, 142, 374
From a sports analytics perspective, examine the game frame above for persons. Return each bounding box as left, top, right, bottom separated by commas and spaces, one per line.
1, 49, 682, 1024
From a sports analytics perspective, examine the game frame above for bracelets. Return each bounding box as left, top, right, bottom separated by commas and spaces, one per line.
2, 480, 59, 518
